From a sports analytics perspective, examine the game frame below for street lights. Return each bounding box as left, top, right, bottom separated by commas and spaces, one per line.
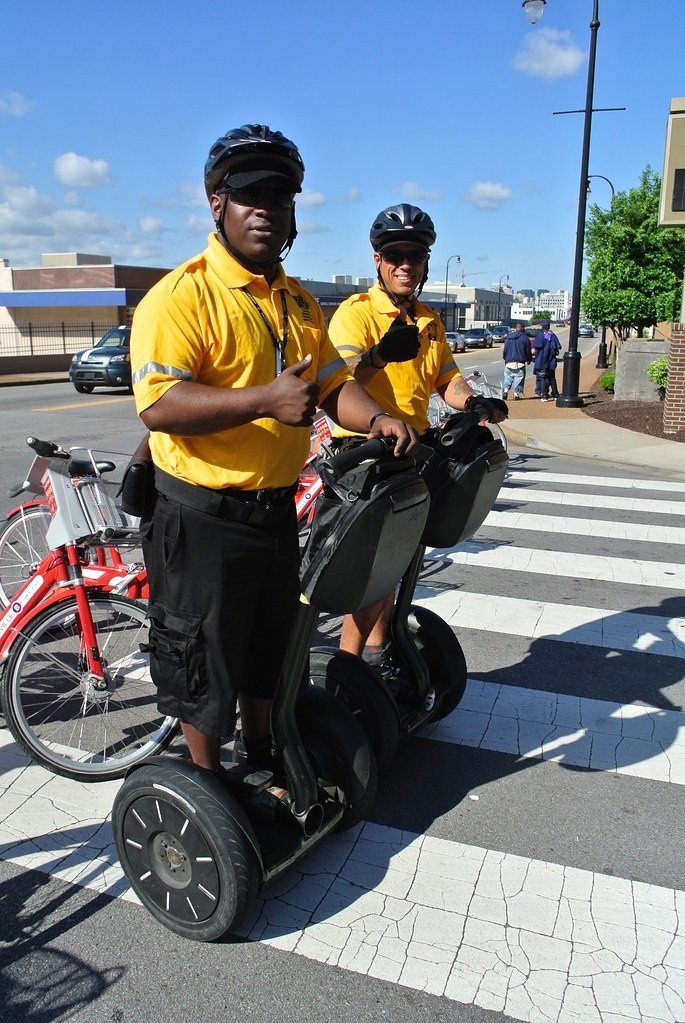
443, 253, 462, 332
524, 1, 626, 407
500, 275, 511, 327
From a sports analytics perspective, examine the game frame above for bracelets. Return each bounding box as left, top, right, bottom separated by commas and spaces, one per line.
370, 411, 394, 428
369, 345, 387, 369
466, 394, 478, 410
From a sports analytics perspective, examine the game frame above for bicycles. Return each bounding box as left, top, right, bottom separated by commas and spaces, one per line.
428, 370, 507, 454
0, 433, 331, 780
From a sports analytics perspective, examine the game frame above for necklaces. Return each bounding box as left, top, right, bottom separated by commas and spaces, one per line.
240, 285, 288, 351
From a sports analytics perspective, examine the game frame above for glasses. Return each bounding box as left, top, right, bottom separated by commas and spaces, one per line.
216, 184, 295, 211
378, 252, 429, 265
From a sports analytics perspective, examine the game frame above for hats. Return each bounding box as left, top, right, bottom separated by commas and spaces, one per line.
220, 166, 302, 193
541, 320, 550, 328
374, 231, 434, 253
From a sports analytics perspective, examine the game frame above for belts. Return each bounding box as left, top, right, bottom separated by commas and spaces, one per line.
210, 481, 299, 505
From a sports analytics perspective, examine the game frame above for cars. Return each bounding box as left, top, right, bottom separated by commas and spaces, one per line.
576, 325, 594, 338
444, 326, 513, 351
69, 323, 135, 393
555, 320, 566, 326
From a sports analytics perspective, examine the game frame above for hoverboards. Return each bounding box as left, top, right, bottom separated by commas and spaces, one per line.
112, 406, 510, 941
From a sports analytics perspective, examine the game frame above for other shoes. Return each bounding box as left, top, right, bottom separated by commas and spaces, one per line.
513, 392, 519, 400
535, 392, 559, 402
214, 767, 260, 801
502, 392, 507, 399
230, 726, 288, 778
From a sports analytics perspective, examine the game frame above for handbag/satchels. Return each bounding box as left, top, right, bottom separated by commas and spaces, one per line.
116, 432, 298, 521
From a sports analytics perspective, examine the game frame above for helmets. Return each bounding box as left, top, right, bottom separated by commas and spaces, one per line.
204, 123, 305, 203
370, 204, 436, 251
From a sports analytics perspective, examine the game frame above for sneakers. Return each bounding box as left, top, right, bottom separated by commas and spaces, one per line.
368, 651, 404, 680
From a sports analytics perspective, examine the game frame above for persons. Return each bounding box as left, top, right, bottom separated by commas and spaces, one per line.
325, 204, 508, 662
531, 322, 563, 403
503, 323, 532, 400
130, 124, 420, 798
